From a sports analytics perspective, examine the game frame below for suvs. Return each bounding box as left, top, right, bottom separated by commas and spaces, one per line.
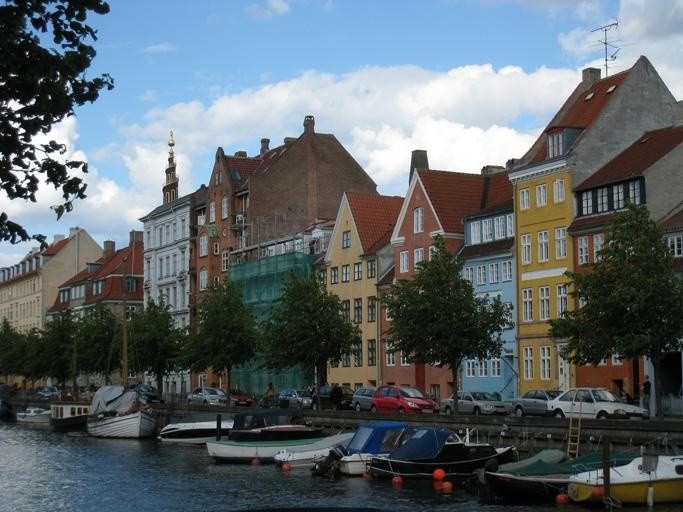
312, 386, 354, 412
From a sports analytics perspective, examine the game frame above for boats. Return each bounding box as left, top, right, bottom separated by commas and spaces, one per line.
314, 422, 417, 475
458, 448, 567, 498
365, 426, 519, 479
158, 420, 233, 444
205, 408, 355, 466
86, 385, 157, 438
49, 401, 92, 429
15, 407, 49, 422
567, 432, 682, 503
38, 409, 51, 418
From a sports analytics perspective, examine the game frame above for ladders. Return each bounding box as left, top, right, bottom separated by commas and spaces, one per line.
567, 403, 582, 461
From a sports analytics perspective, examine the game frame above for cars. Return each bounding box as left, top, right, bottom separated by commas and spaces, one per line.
184, 384, 312, 409
130, 384, 157, 400
35, 385, 60, 400
512, 389, 563, 418
351, 387, 374, 411
441, 391, 507, 415
546, 387, 649, 421
370, 385, 439, 415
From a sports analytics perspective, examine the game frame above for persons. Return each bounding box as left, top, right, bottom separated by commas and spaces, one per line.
640, 375, 651, 416
265, 381, 275, 397
310, 382, 344, 411
